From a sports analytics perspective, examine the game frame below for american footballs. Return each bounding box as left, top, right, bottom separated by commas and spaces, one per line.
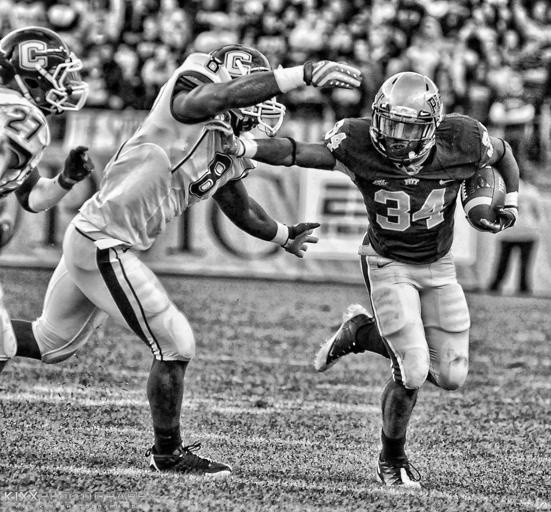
461, 163, 507, 231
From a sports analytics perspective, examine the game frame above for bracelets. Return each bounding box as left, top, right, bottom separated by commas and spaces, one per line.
270, 220, 290, 246
504, 192, 520, 207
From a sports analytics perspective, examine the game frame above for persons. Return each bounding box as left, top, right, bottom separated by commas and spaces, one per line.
1, 1, 550, 163
485, 165, 543, 293
11, 45, 363, 478
0, 27, 93, 373
205, 72, 520, 489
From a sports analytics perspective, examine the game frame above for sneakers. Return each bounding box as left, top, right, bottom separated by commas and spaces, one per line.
375, 452, 421, 491
313, 304, 375, 373
146, 440, 233, 476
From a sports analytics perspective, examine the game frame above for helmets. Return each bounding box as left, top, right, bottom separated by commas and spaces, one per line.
0, 26, 89, 115
368, 71, 442, 166
208, 44, 286, 137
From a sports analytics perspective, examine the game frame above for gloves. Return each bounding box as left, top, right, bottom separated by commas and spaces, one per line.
203, 119, 258, 159
480, 191, 517, 234
58, 147, 95, 191
302, 59, 363, 89
281, 223, 320, 258
